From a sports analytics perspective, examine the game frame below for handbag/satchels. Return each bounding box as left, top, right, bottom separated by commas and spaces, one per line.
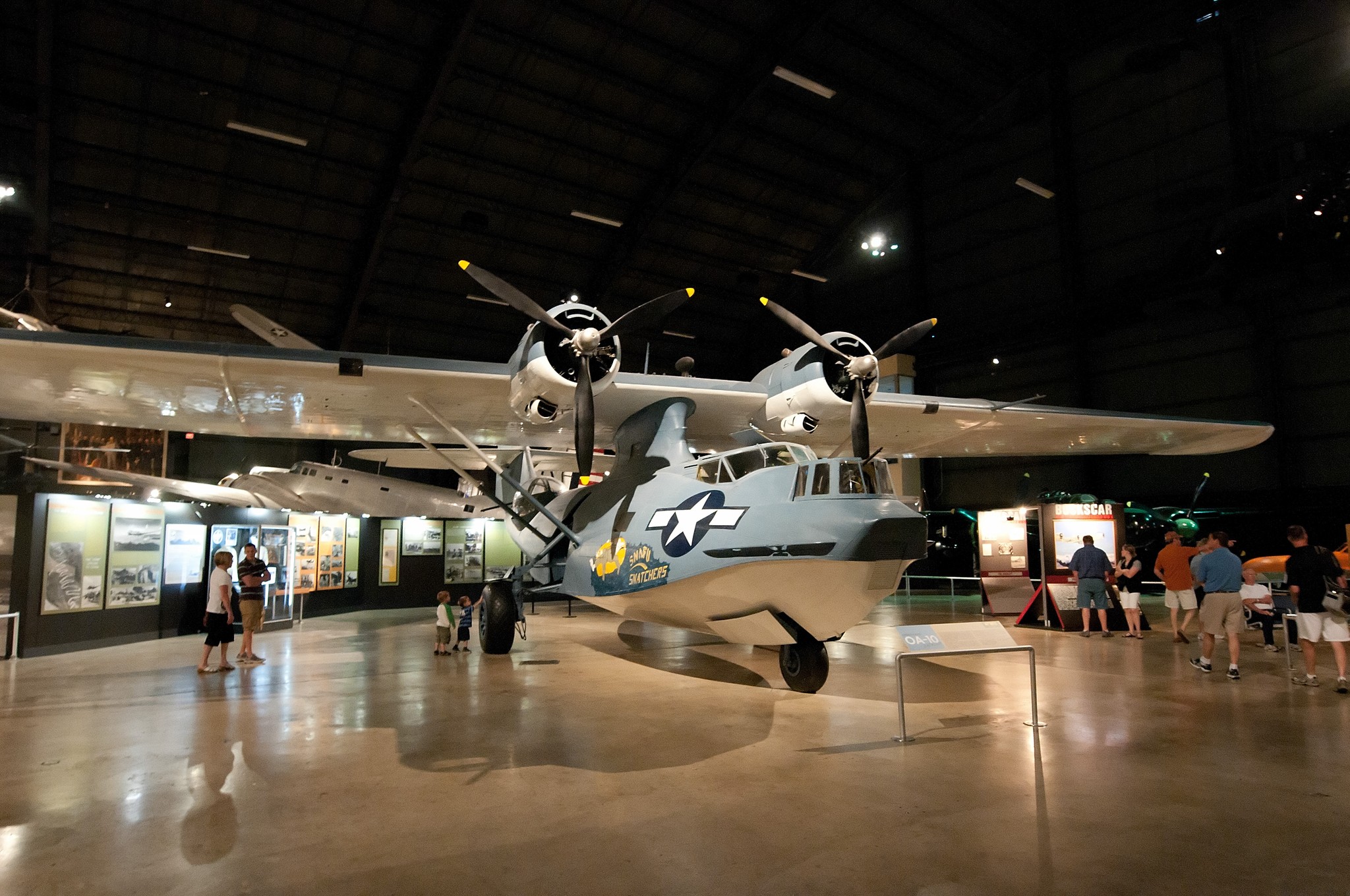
1322, 583, 1350, 618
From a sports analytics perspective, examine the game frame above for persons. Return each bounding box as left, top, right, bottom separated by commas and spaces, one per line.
1153, 531, 1210, 645
235, 543, 271, 664
1068, 536, 1114, 637
1189, 532, 1247, 679
1285, 524, 1350, 692
1239, 568, 1279, 652
197, 552, 236, 673
433, 591, 455, 656
452, 595, 483, 653
1190, 537, 1225, 641
1115, 544, 1145, 639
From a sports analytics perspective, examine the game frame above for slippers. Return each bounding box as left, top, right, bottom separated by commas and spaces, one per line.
1137, 632, 1143, 639
219, 663, 235, 671
1122, 631, 1135, 638
198, 666, 218, 673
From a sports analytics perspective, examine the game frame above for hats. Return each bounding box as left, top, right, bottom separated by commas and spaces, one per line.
1165, 531, 1184, 540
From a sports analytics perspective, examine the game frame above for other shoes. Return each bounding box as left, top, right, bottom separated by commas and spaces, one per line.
462, 648, 470, 652
1178, 628, 1190, 644
1173, 636, 1183, 643
452, 645, 460, 651
1102, 631, 1114, 637
440, 650, 451, 655
434, 650, 439, 654
1079, 631, 1091, 637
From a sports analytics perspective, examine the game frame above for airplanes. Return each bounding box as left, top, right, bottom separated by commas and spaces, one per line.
0, 260, 1276, 694
917, 470, 1274, 594
1239, 524, 1350, 585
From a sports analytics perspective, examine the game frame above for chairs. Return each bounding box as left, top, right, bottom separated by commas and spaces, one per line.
1242, 602, 1287, 650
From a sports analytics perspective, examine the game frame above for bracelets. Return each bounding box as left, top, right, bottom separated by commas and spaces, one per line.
1256, 599, 1259, 603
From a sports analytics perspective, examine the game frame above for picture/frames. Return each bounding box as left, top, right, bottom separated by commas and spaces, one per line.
56, 423, 170, 489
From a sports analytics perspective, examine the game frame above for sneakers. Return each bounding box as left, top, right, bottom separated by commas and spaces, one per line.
1264, 644, 1280, 652
244, 654, 266, 664
1189, 658, 1211, 672
1290, 643, 1303, 652
1226, 668, 1240, 678
237, 652, 246, 660
1291, 675, 1319, 686
1337, 679, 1348, 692
